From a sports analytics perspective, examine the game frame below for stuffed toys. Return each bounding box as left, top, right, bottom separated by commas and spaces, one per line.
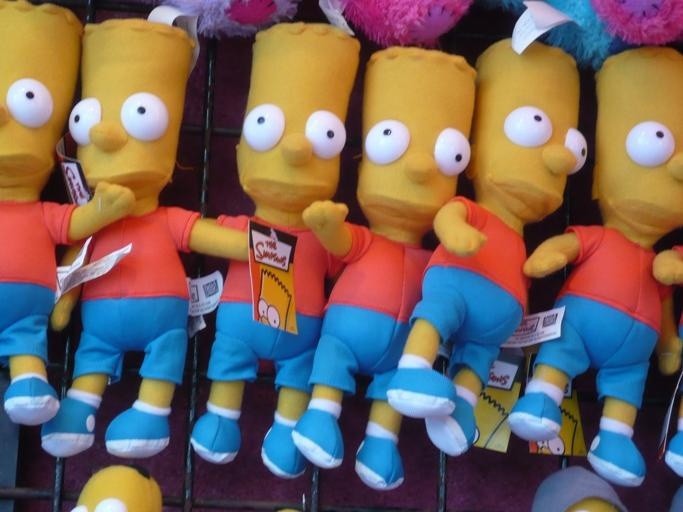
0, 0, 682, 511
